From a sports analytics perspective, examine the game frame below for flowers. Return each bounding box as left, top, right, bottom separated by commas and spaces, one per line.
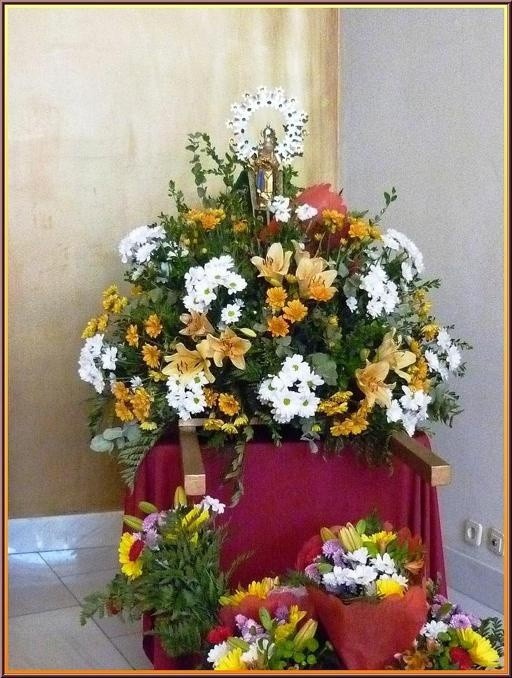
204, 574, 322, 674
109, 492, 228, 578
76, 130, 472, 511
386, 591, 505, 672
305, 516, 415, 600
